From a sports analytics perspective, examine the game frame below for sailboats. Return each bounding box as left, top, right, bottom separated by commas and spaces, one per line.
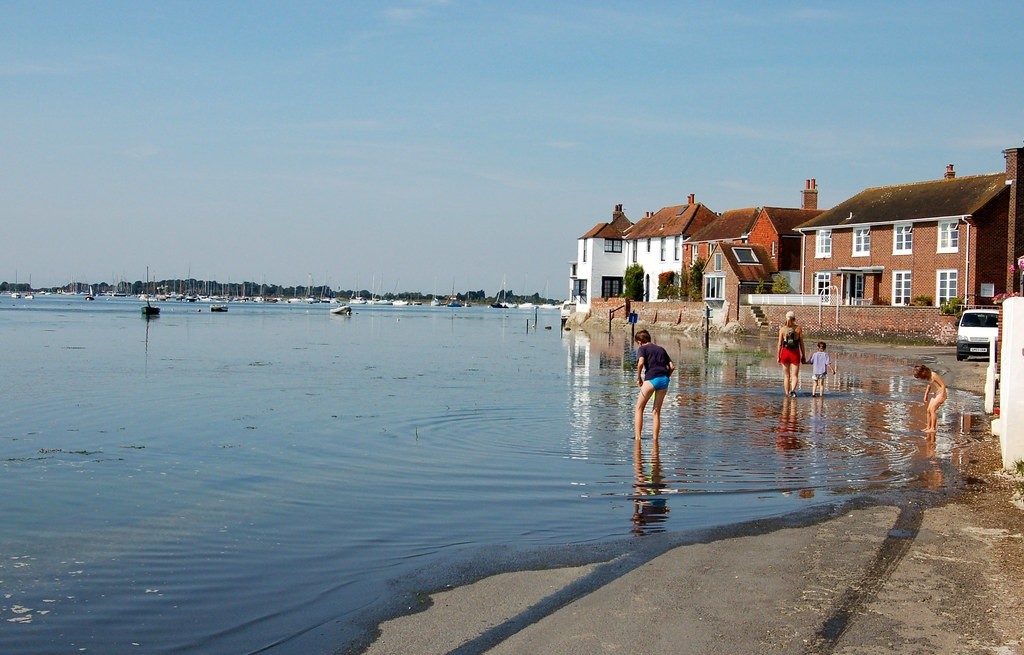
11, 268, 564, 310
140, 266, 160, 315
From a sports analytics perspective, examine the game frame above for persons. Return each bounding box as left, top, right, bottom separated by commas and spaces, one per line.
805, 341, 836, 396
776, 311, 805, 397
634, 329, 675, 441
913, 364, 947, 432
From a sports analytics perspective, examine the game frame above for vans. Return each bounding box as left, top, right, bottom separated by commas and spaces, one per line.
954, 308, 999, 361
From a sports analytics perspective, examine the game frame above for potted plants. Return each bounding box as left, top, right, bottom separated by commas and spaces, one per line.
911, 293, 935, 306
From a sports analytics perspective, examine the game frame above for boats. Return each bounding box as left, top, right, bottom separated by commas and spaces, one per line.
210, 304, 229, 313
329, 303, 351, 315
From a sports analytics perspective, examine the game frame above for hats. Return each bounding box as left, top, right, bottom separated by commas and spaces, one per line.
817, 342, 826, 348
785, 311, 795, 319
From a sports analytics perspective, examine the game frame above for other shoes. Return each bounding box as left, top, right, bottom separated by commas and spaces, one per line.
790, 391, 797, 397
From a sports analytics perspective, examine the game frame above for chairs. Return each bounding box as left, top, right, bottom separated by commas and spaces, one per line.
967, 315, 980, 326
985, 317, 998, 327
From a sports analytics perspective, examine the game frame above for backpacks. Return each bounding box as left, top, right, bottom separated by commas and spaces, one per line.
781, 325, 800, 349
